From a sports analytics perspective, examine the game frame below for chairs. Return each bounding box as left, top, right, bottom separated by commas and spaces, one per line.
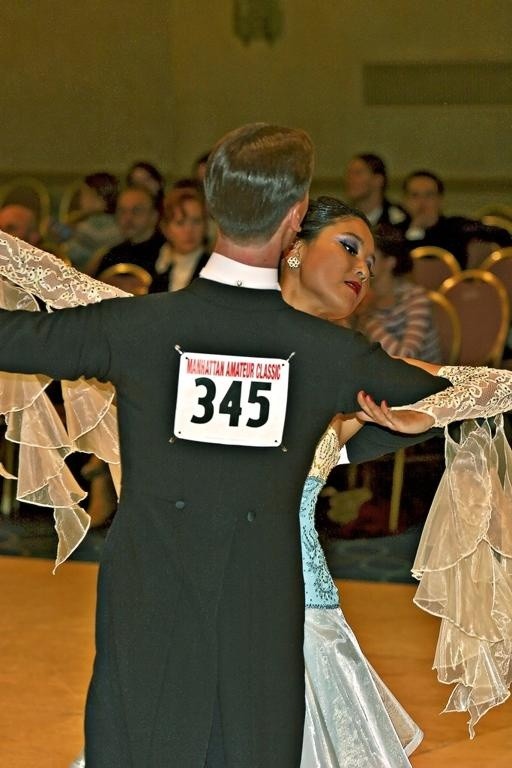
0, 176, 511, 531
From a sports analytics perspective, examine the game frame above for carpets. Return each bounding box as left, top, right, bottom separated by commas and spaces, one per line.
0, 504, 423, 587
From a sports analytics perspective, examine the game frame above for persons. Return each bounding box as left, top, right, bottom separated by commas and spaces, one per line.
1, 123, 512, 768
